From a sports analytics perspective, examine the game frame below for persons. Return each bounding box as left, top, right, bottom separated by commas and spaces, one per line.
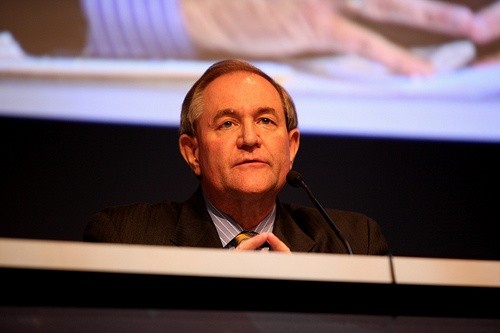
92, 58, 391, 256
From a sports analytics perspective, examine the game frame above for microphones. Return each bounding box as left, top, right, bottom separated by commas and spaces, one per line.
286, 171, 353, 255
